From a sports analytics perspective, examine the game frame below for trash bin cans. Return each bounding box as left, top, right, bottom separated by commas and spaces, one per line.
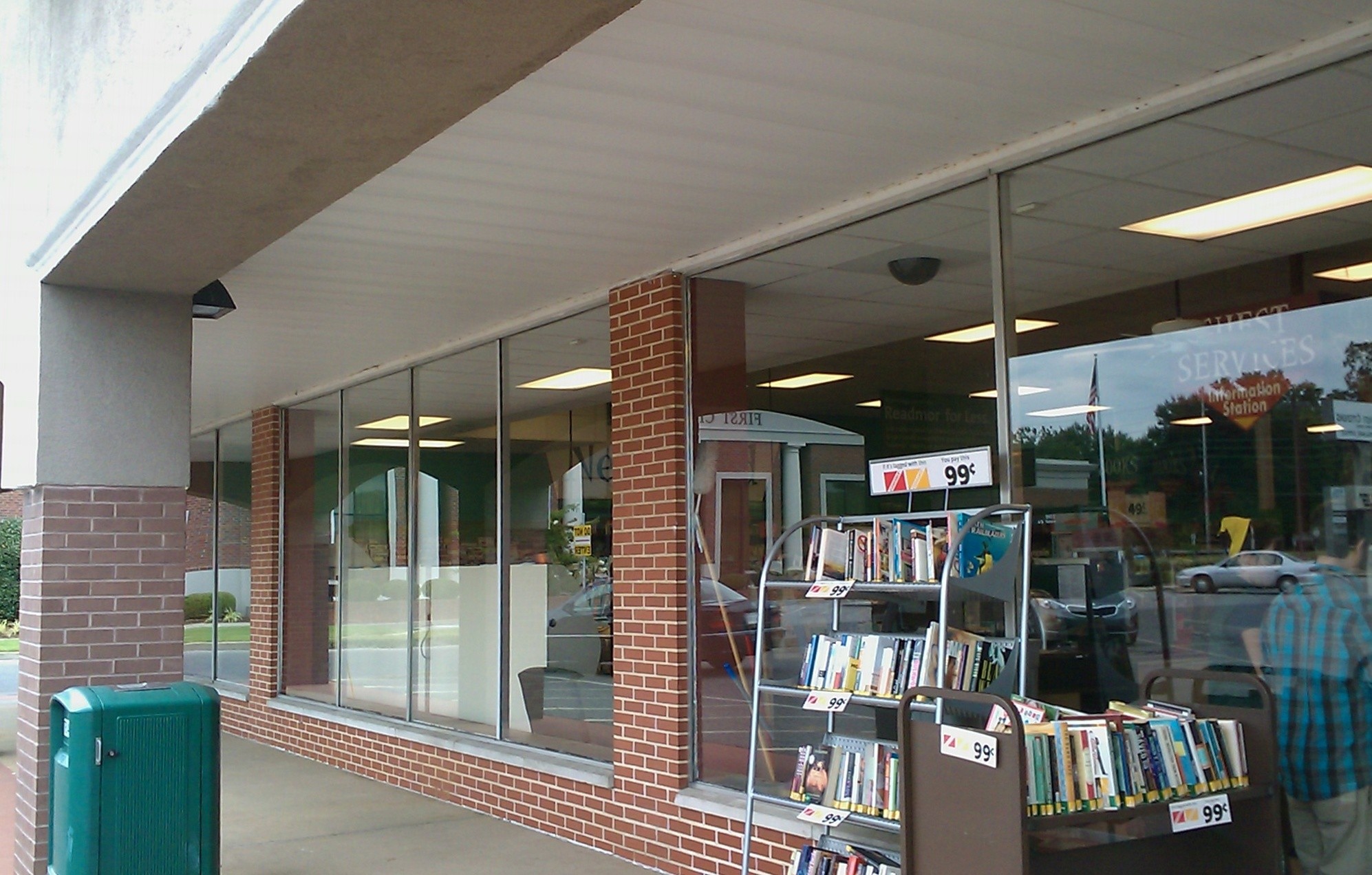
46, 681, 220, 874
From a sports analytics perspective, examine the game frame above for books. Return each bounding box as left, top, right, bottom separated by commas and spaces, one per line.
787, 513, 1247, 875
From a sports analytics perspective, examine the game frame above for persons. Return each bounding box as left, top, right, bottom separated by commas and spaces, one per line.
1208, 506, 1372, 875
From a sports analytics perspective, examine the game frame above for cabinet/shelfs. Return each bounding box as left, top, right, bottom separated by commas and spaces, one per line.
898, 668, 1292, 875
742, 506, 1023, 875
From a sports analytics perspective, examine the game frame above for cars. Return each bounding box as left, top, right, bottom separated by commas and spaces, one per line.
548, 573, 786, 675
1175, 548, 1319, 596
1029, 591, 1142, 647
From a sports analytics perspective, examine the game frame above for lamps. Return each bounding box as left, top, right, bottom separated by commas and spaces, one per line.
192, 279, 237, 320
